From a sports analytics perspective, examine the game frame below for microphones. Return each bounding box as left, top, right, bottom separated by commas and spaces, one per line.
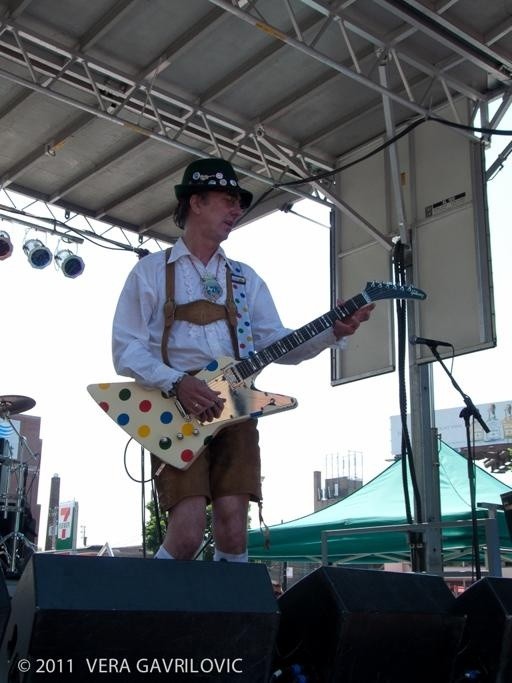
409, 334, 452, 346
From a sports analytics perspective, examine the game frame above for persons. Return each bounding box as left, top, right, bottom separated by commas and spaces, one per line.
111, 158, 375, 563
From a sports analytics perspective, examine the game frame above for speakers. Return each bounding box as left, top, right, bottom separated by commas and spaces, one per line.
0, 552, 282, 683
277, 564, 460, 682
457, 575, 512, 683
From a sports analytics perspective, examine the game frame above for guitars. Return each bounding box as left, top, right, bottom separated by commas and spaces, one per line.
87, 280, 426, 472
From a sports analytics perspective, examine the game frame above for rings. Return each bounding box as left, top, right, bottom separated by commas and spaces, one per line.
193, 403, 199, 408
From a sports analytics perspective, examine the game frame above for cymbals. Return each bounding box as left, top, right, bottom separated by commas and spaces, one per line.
0, 395, 35, 417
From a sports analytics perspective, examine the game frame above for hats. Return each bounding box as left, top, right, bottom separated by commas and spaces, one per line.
175, 158, 253, 209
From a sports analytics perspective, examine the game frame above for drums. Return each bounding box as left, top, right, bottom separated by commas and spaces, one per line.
1, 437, 14, 520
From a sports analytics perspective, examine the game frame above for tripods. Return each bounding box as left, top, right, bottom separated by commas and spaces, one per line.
0, 417, 41, 578
